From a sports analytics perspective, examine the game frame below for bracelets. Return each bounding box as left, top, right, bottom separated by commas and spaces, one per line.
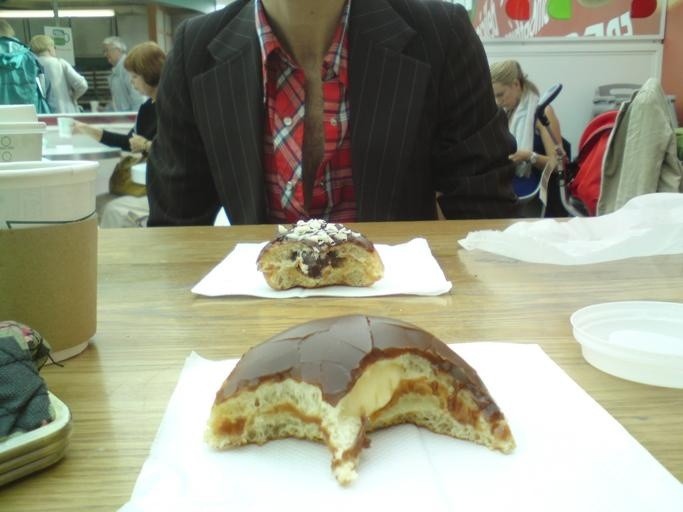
140, 136, 150, 156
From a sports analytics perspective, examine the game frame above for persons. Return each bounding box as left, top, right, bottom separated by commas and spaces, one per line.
0, 21, 49, 115
101, 36, 148, 112
72, 42, 168, 228
28, 35, 87, 114
143, 0, 519, 226
435, 60, 571, 221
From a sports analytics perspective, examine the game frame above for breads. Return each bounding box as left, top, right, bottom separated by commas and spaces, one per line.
256, 216, 384, 292
209, 314, 513, 486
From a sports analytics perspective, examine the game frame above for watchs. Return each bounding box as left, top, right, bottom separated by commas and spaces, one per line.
528, 152, 538, 165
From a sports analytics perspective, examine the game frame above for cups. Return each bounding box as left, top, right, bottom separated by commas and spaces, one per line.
0, 104, 101, 366
